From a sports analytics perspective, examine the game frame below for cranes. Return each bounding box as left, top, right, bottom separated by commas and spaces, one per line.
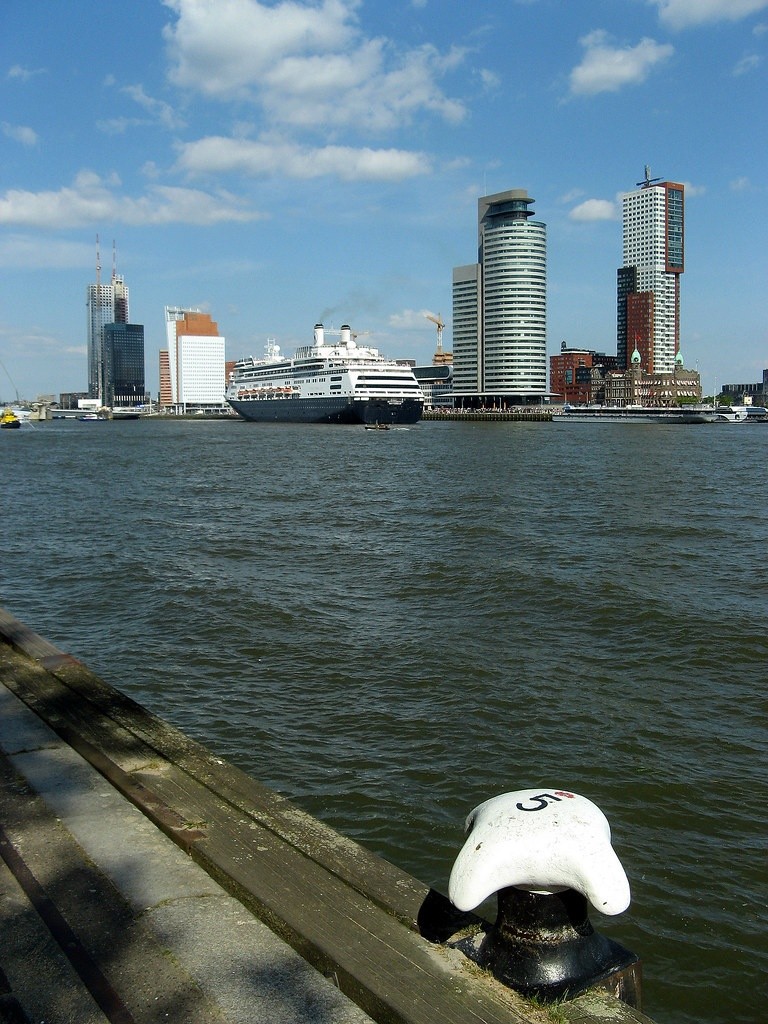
426, 311, 445, 365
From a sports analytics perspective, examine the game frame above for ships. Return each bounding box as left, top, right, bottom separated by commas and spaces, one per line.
223, 322, 426, 425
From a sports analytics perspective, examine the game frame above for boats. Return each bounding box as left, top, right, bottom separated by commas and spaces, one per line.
238, 387, 301, 398
0, 405, 22, 429
552, 406, 749, 424
78, 412, 109, 422
